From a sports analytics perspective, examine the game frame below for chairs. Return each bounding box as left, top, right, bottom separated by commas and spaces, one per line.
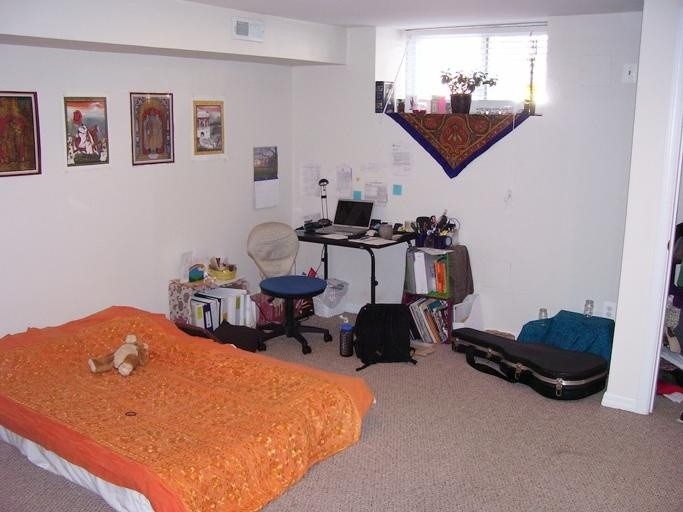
246, 221, 329, 344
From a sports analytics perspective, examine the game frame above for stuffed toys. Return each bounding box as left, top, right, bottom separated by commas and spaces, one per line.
86, 330, 149, 377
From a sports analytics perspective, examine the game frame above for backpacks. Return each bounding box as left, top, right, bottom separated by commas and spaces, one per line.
352, 303, 417, 371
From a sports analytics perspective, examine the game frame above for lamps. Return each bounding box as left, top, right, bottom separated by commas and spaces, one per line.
318, 178, 333, 226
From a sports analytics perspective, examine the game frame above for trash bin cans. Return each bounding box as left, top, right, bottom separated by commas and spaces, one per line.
312, 280, 349, 318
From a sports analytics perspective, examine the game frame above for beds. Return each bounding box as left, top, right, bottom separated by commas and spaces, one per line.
0, 303, 381, 511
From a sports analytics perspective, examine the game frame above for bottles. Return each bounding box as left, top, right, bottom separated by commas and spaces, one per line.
430, 95, 438, 114
339, 324, 353, 357
583, 299, 593, 318
537, 308, 547, 319
378, 223, 393, 239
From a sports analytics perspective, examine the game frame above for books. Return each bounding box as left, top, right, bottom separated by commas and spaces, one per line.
190, 286, 259, 333
403, 249, 448, 345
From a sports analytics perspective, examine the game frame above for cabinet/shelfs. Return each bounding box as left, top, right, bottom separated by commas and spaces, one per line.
402, 247, 468, 348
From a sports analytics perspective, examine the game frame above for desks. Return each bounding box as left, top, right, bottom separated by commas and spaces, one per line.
292, 223, 416, 306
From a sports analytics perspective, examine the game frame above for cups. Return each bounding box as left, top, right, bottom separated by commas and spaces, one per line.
414, 234, 425, 247
434, 235, 452, 249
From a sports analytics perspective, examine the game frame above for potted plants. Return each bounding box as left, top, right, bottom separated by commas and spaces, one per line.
438, 62, 498, 114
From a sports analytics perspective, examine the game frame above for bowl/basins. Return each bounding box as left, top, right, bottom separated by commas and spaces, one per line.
207, 268, 236, 281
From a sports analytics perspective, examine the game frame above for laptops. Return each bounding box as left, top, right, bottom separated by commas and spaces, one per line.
315, 199, 374, 239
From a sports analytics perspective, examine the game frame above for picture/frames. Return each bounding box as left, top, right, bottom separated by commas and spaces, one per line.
61, 95, 109, 167
0, 91, 42, 180
190, 101, 224, 156
126, 92, 175, 168
